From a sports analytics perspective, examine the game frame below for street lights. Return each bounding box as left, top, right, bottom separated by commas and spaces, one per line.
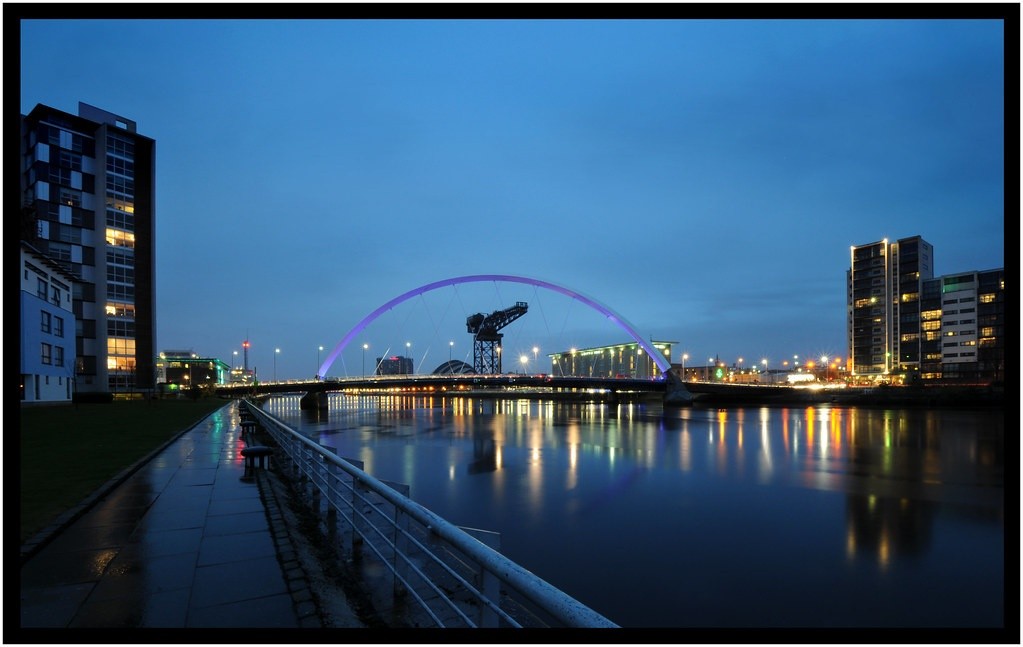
821, 356, 828, 384
682, 354, 687, 380
231, 351, 238, 371
362, 344, 368, 377
762, 359, 768, 386
570, 348, 576, 377
496, 347, 501, 374
449, 341, 454, 374
317, 346, 323, 380
706, 357, 713, 379
273, 348, 280, 382
405, 343, 410, 377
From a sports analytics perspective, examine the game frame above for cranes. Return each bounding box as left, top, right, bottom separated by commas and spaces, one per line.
466, 299, 528, 376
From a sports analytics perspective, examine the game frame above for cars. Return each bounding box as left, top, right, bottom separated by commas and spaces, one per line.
810, 376, 874, 388
